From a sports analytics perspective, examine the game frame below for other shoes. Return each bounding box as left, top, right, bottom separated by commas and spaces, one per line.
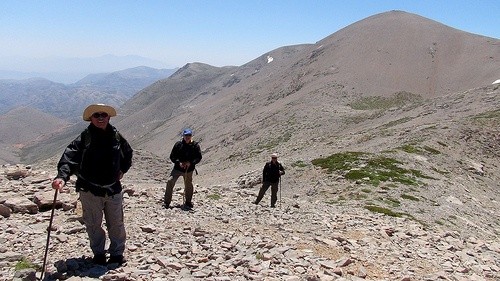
252, 202, 258, 205
185, 203, 194, 208
110, 254, 124, 266
162, 203, 169, 209
270, 206, 276, 208
93, 254, 106, 266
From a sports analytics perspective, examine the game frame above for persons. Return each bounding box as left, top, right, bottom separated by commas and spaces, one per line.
162, 130, 202, 212
251, 153, 286, 208
52, 104, 133, 267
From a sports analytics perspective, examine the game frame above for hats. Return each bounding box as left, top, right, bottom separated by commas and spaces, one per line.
82, 103, 117, 121
181, 128, 192, 136
270, 153, 278, 158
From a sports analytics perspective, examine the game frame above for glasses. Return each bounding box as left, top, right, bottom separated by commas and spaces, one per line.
92, 112, 109, 118
